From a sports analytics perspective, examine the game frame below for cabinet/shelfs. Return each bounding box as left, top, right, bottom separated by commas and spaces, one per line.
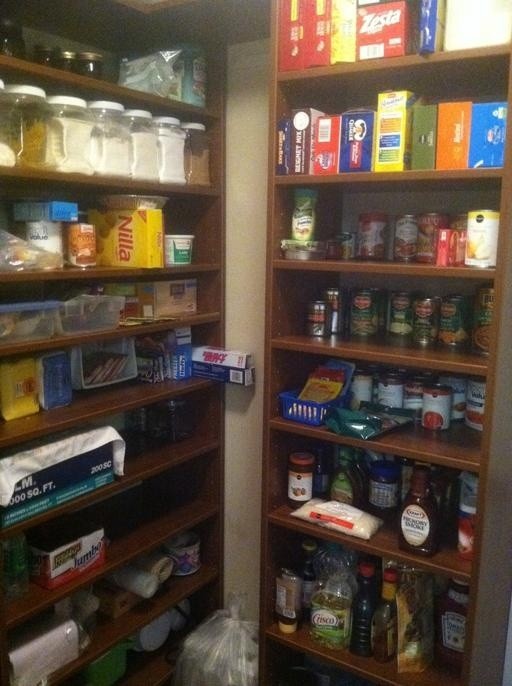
0, 41, 223, 686
260, 0, 512, 686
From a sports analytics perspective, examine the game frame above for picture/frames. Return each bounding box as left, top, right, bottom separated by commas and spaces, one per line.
87, 208, 164, 268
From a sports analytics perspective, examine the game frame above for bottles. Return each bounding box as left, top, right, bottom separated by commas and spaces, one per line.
180, 122, 214, 185
38, 44, 59, 69
388, 290, 419, 339
330, 471, 354, 504
325, 237, 341, 261
412, 297, 442, 352
347, 371, 375, 412
420, 385, 451, 429
89, 101, 133, 177
313, 441, 330, 498
321, 286, 346, 334
125, 109, 160, 182
274, 562, 302, 635
339, 232, 358, 259
60, 50, 79, 72
368, 459, 399, 518
286, 450, 315, 509
415, 210, 446, 266
467, 286, 501, 359
357, 213, 389, 261
298, 540, 319, 621
377, 374, 404, 413
467, 380, 490, 433
437, 293, 468, 352
395, 464, 436, 556
345, 286, 379, 340
306, 299, 325, 338
371, 565, 401, 663
457, 500, 481, 564
393, 214, 419, 262
80, 52, 105, 78
404, 378, 427, 417
0, 18, 25, 57
1, 86, 63, 170
351, 563, 379, 661
45, 95, 97, 176
151, 116, 187, 183
434, 575, 477, 672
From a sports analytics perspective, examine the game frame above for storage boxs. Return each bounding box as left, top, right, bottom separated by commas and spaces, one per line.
29, 517, 106, 591
0, 425, 115, 530
0, 302, 61, 342
62, 295, 121, 335
